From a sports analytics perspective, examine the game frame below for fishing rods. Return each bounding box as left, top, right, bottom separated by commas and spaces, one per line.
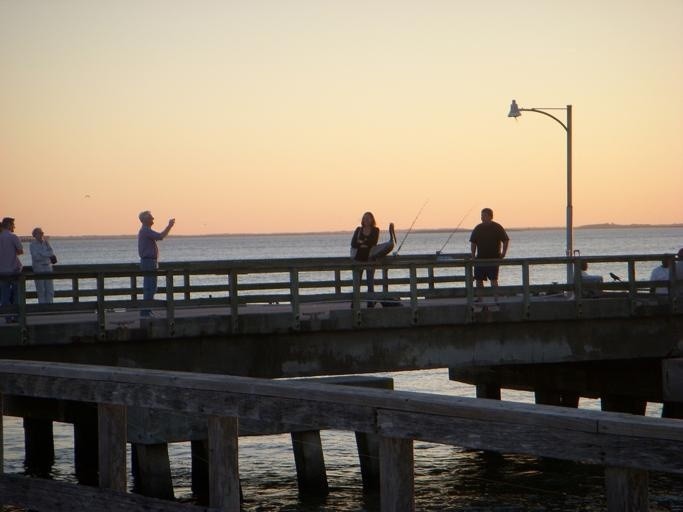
393, 197, 429, 256
437, 209, 473, 255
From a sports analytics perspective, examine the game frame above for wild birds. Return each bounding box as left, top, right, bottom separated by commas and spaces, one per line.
367, 222, 396, 257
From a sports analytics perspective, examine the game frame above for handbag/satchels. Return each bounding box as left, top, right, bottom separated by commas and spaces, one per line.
349, 246, 357, 260
49, 254, 57, 264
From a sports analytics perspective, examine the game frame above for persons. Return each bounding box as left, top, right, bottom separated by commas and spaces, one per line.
29, 227, 56, 303
0, 218, 24, 324
137, 210, 175, 317
469, 208, 509, 302
581, 260, 591, 277
350, 212, 378, 307
649, 253, 670, 294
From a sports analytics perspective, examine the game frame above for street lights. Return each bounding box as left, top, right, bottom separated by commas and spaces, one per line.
507, 98, 574, 299
